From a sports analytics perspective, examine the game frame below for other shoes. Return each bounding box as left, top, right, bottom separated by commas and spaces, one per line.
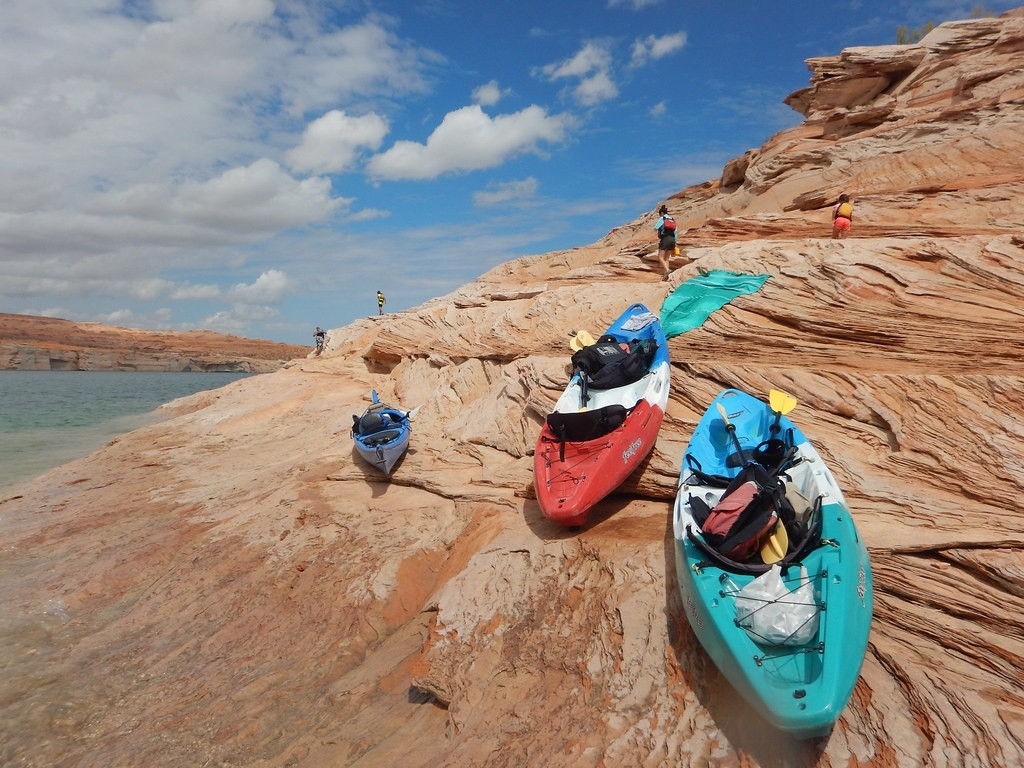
662, 270, 671, 281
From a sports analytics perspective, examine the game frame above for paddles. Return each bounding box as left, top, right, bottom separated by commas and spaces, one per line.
715, 389, 797, 565
569, 329, 596, 414
395, 403, 424, 422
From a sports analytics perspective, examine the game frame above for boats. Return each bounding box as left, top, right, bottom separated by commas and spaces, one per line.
532, 301, 671, 527
353, 400, 414, 473
673, 388, 873, 739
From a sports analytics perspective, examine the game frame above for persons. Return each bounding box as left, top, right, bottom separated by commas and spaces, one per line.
377, 291, 387, 314
654, 206, 677, 281
313, 327, 327, 355
832, 195, 852, 239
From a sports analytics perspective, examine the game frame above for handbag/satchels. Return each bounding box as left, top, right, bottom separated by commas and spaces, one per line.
735, 563, 817, 647
703, 463, 797, 558
671, 245, 680, 257
